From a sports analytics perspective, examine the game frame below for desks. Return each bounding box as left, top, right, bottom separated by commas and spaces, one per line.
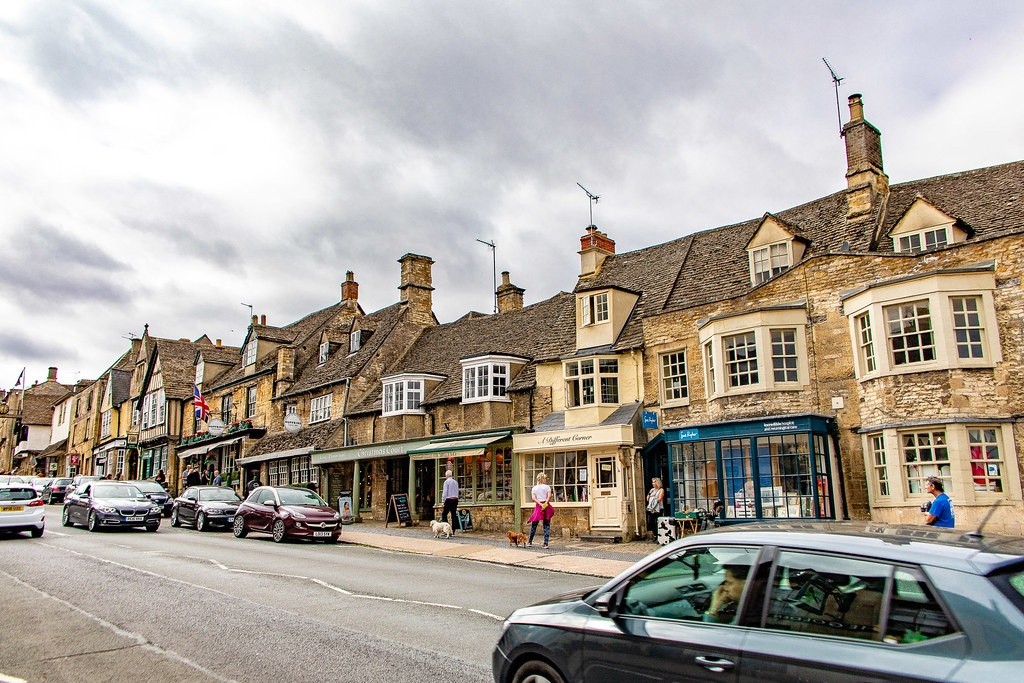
674, 518, 699, 539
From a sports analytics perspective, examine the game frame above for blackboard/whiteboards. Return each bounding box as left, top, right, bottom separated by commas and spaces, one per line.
386, 493, 412, 524
456, 509, 474, 530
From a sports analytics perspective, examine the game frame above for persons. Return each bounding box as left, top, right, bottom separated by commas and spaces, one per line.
703, 564, 751, 624
182, 465, 210, 489
923, 476, 955, 528
700, 458, 718, 509
526, 472, 554, 549
38, 468, 45, 476
342, 502, 351, 514
789, 568, 825, 604
114, 473, 120, 480
442, 470, 459, 535
155, 469, 165, 484
246, 476, 262, 498
107, 474, 112, 480
0, 467, 18, 475
212, 470, 221, 486
647, 478, 664, 541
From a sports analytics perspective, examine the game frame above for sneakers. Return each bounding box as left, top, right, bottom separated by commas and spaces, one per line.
526, 542, 532, 546
542, 544, 549, 549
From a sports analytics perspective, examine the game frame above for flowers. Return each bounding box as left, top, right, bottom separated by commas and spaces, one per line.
190, 455, 201, 463
227, 423, 239, 433
240, 420, 252, 430
204, 452, 217, 464
182, 431, 215, 444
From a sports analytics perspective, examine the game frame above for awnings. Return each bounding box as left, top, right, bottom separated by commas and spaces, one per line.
407, 434, 507, 460
35, 439, 67, 458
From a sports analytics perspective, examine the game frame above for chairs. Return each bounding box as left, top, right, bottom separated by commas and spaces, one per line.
6, 490, 21, 499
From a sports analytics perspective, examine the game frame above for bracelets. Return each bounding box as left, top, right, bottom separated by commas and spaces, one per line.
704, 611, 718, 616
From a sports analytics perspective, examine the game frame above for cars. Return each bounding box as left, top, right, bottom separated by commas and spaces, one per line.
62, 480, 161, 532
171, 485, 243, 532
118, 480, 175, 517
0, 483, 46, 538
0, 475, 107, 505
492, 519, 1024, 683
233, 486, 343, 543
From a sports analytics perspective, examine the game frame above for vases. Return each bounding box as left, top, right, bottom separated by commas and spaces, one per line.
233, 427, 239, 431
247, 422, 253, 428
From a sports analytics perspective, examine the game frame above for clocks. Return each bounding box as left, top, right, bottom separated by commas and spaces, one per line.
0, 404, 9, 414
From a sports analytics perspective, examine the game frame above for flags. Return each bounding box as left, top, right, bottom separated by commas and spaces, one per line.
194, 386, 209, 424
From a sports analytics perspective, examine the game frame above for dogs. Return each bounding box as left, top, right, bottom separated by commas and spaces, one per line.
429, 520, 453, 539
506, 531, 528, 548
